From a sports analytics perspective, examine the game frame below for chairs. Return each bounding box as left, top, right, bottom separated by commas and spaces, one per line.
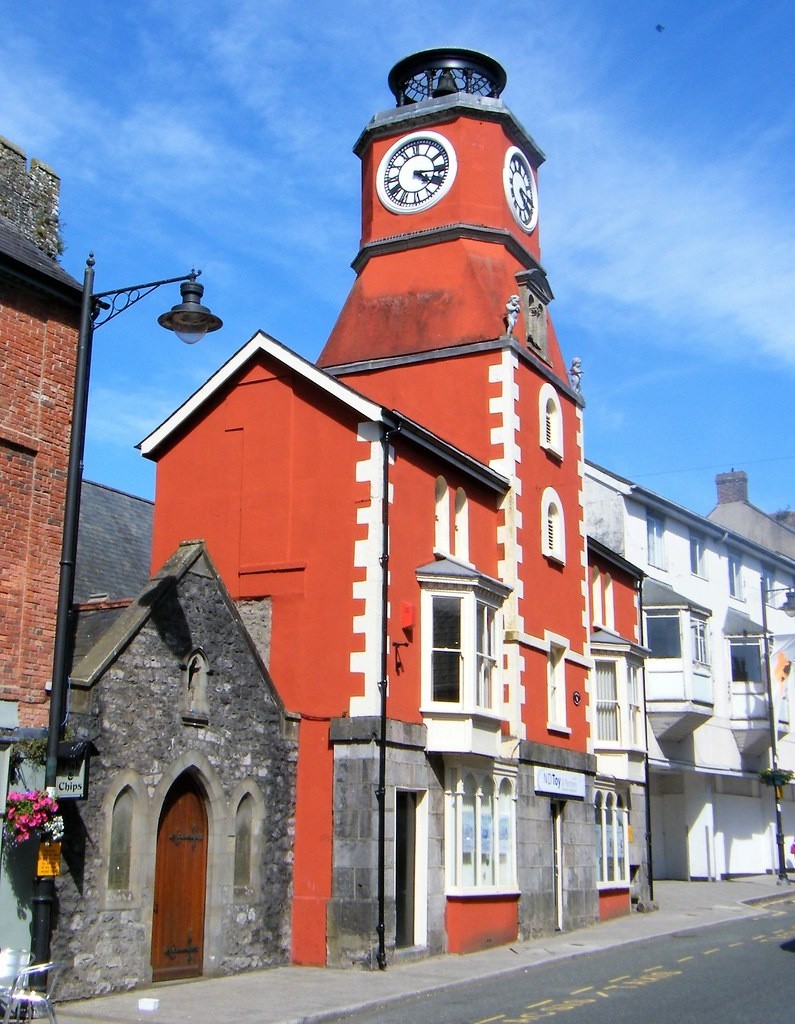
0, 947, 74, 1024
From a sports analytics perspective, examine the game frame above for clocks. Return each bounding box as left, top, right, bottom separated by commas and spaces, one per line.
376, 131, 457, 213
503, 140, 538, 235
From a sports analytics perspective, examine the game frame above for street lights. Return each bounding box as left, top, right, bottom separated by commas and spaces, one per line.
761, 575, 795, 886
24, 249, 223, 1021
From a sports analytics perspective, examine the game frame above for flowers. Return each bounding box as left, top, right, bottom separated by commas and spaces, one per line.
2, 786, 69, 853
